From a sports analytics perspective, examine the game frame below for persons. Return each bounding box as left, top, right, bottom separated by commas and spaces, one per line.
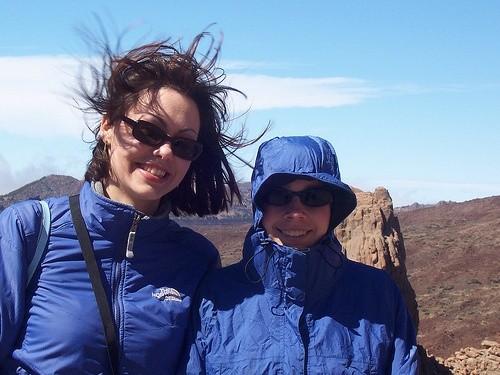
0, 20, 243, 375
181, 136, 421, 375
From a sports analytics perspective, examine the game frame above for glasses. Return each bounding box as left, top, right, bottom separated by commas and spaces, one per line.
261, 183, 333, 208
117, 113, 204, 162
